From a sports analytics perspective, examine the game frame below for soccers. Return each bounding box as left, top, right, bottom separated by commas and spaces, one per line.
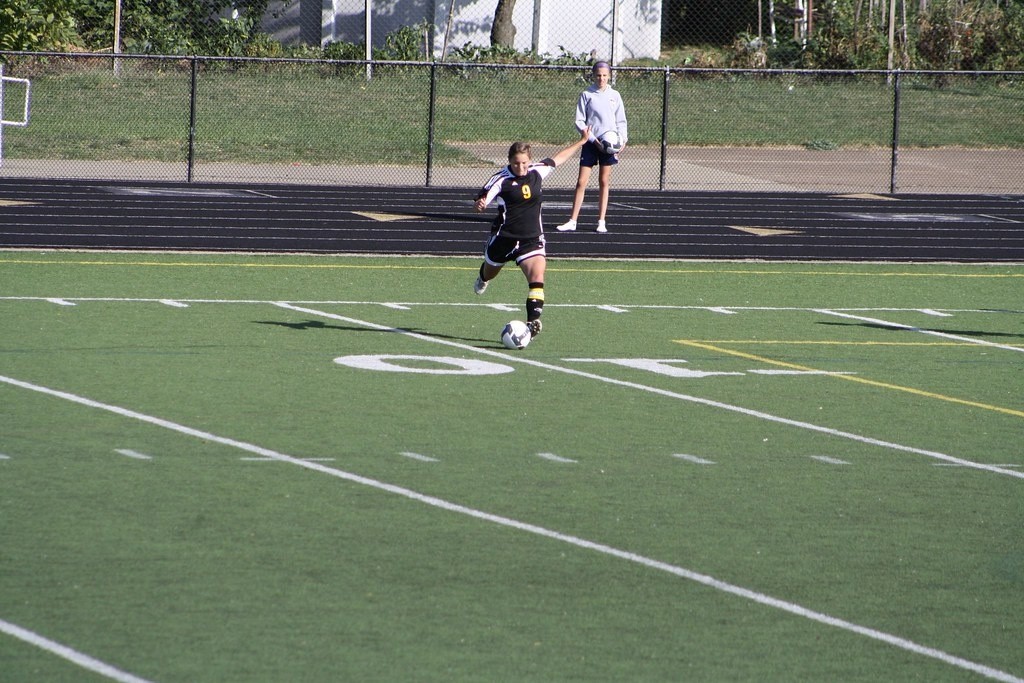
598, 130, 624, 154
501, 320, 532, 350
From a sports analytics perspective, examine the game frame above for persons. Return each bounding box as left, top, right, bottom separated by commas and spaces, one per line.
556, 61, 628, 233
473, 125, 592, 342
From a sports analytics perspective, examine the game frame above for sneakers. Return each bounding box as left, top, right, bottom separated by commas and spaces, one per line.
526, 319, 542, 343
474, 268, 489, 295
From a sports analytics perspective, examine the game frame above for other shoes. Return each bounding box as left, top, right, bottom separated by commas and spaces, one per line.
557, 219, 576, 231
597, 220, 607, 232
